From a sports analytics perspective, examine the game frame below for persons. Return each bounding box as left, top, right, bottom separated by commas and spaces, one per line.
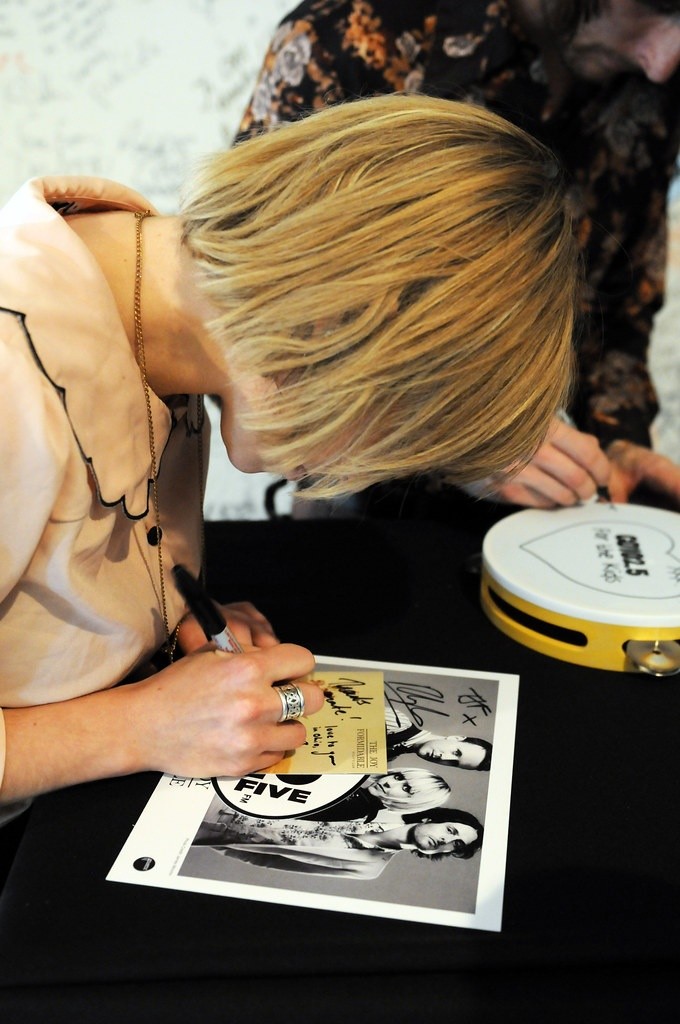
230, 0, 679, 519
0, 97, 575, 808
195, 703, 493, 876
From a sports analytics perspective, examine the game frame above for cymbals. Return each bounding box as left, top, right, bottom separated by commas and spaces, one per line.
627, 641, 680, 676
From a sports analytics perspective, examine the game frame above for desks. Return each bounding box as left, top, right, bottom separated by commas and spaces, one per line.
0, 519, 680, 1024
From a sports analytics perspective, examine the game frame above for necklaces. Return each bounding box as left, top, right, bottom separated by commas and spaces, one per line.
130, 210, 203, 669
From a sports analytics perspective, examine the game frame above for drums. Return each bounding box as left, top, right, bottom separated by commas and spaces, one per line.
480, 501, 680, 672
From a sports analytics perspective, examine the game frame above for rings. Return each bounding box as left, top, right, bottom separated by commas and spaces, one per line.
273, 683, 305, 724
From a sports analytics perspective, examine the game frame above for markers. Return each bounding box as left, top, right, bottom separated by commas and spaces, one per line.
555, 406, 614, 508
170, 564, 241, 657
